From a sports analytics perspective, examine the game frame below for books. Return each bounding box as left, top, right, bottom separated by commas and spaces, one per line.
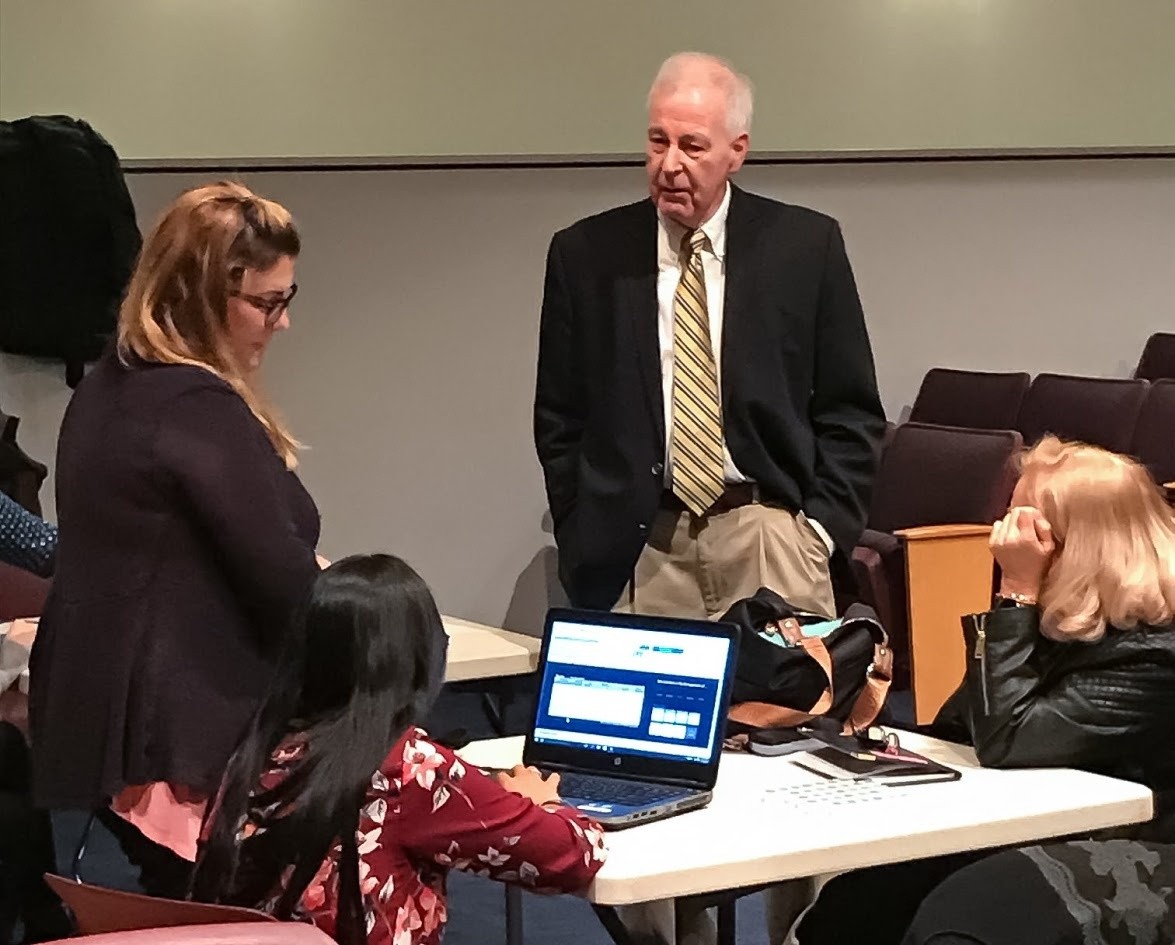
787, 730, 963, 787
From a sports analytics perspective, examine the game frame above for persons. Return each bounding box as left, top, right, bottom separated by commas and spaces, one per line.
783, 433, 1175, 945
25, 179, 472, 936
191, 553, 607, 945
531, 48, 888, 945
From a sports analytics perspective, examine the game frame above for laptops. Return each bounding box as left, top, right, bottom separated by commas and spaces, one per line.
529, 607, 744, 830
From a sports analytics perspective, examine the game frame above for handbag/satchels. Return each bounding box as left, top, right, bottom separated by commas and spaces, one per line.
719, 588, 901, 755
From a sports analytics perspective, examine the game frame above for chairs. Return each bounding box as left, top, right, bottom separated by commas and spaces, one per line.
0, 506, 570, 945
848, 332, 1175, 720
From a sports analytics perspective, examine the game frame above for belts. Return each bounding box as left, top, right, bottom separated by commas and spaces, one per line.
662, 483, 768, 515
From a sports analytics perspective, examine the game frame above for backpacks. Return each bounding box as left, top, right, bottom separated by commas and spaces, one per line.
0, 114, 143, 388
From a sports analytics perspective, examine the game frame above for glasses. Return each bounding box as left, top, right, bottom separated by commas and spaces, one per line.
221, 283, 299, 324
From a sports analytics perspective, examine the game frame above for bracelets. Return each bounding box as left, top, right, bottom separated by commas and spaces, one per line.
995, 592, 1038, 602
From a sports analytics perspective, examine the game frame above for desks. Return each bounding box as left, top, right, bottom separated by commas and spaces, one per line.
0, 611, 545, 738
451, 710, 1152, 945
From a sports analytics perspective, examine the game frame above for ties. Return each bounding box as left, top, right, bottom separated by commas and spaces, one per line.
673, 230, 725, 517
